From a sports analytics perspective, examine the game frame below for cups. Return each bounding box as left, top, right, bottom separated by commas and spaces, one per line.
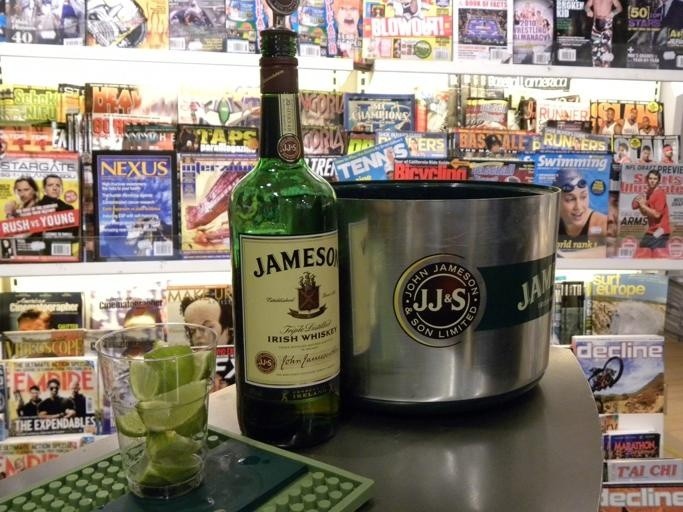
95, 322, 218, 500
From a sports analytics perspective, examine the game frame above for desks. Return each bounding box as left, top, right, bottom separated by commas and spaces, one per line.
0, 345, 605, 512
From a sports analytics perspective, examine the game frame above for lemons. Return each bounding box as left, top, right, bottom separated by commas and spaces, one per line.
114, 344, 217, 485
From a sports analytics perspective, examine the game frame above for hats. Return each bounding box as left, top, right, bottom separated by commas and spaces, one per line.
551, 167, 582, 189
484, 133, 502, 149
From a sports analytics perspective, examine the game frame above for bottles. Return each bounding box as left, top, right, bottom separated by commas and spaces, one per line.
226, 27, 345, 452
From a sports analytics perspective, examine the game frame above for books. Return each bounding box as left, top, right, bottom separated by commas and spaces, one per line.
0, 0, 272, 54
0, 81, 261, 262
551, 271, 683, 512
0, 284, 234, 479
292, 1, 683, 70
298, 83, 683, 260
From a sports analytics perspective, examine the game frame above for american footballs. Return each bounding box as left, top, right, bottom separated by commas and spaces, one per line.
632, 192, 647, 209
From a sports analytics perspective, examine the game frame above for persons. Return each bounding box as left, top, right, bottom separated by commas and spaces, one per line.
331, 0, 362, 56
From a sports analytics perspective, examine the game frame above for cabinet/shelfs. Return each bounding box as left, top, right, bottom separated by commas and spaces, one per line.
0, 40, 683, 277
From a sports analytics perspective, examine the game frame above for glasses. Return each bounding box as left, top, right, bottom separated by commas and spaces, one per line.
50, 386, 59, 391
562, 178, 587, 193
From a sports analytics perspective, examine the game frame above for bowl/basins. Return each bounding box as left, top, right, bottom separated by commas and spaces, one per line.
335, 181, 562, 407
